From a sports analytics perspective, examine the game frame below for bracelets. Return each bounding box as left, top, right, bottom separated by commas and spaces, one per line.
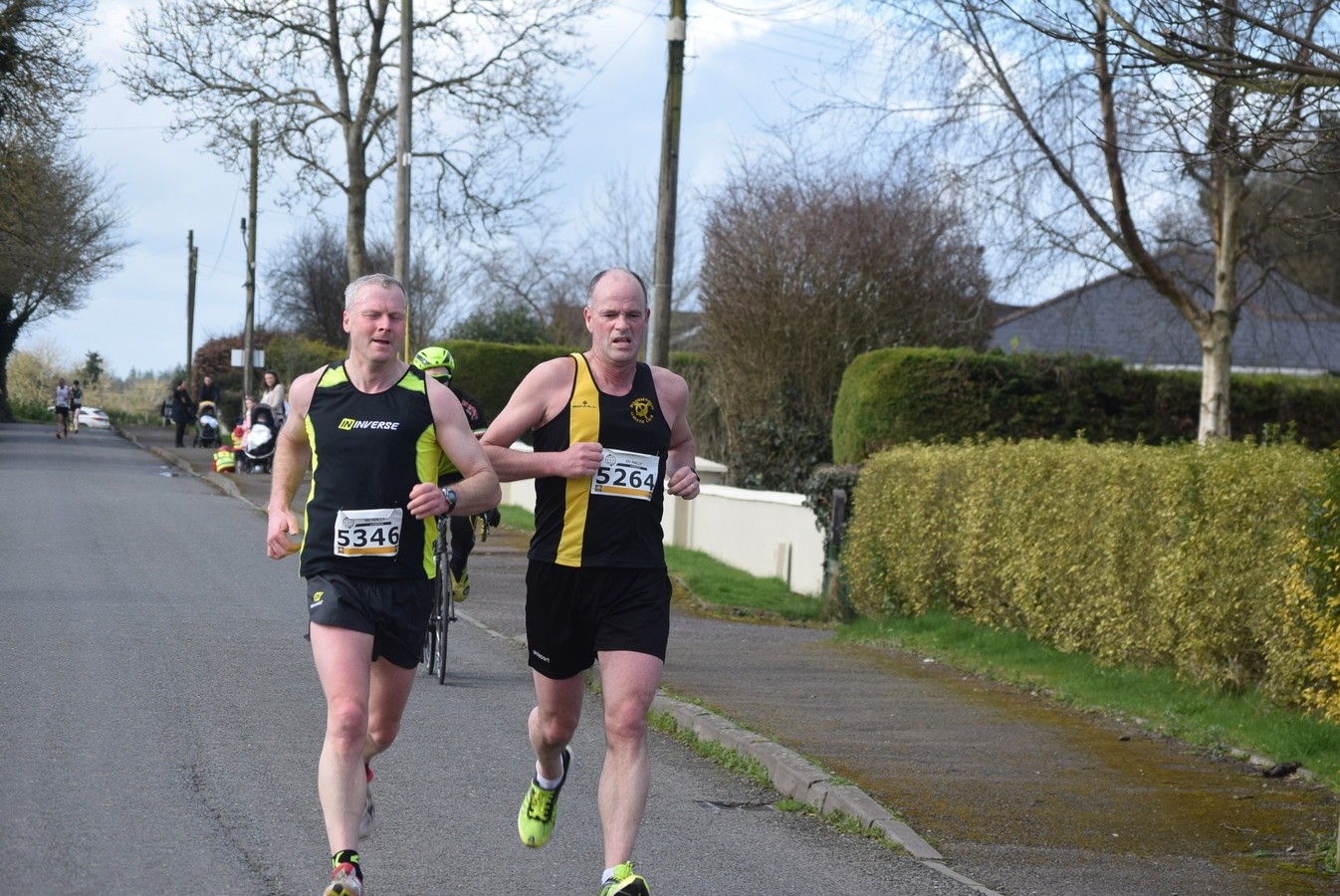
689, 465, 700, 483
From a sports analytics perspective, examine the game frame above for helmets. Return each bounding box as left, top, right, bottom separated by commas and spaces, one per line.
414, 347, 454, 373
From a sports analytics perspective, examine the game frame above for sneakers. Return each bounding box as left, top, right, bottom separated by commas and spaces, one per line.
323, 863, 363, 896
600, 864, 650, 896
360, 773, 374, 838
517, 745, 574, 849
450, 563, 470, 602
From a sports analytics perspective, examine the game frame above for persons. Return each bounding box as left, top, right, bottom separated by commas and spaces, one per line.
54, 377, 82, 439
412, 347, 487, 601
265, 276, 503, 896
172, 371, 285, 474
478, 269, 702, 896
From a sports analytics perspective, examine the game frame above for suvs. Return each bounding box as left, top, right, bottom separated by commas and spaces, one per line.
47, 405, 111, 429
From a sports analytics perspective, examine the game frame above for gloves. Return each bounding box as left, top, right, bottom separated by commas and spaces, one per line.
478, 508, 501, 528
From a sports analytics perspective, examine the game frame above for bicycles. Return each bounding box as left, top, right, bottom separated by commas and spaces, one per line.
426, 511, 489, 683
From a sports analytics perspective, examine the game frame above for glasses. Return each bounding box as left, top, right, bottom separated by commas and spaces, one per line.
429, 374, 452, 383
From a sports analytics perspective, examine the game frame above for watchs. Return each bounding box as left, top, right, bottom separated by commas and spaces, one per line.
439, 485, 458, 519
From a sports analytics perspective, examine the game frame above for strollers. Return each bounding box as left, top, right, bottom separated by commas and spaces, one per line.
242, 403, 280, 475
193, 401, 223, 448
